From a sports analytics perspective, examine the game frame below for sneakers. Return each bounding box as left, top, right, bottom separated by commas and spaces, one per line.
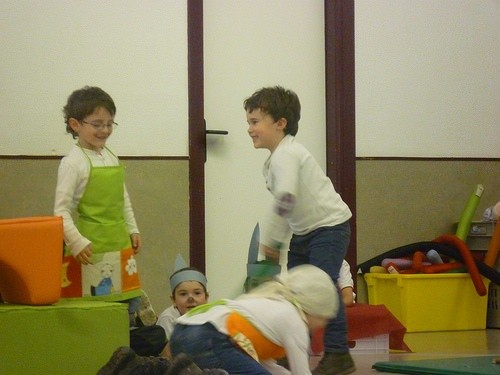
311, 352, 356, 375
277, 356, 288, 370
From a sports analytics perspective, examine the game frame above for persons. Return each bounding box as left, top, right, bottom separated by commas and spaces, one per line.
244, 86, 356, 375
96, 258, 356, 375
53, 86, 142, 314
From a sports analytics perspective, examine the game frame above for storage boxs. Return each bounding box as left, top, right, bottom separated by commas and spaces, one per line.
363, 272, 490, 333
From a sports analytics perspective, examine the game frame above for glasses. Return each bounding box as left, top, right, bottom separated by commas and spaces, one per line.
78, 118, 118, 131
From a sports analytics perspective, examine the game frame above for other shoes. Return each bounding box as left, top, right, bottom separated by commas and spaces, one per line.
348, 341, 356, 348
